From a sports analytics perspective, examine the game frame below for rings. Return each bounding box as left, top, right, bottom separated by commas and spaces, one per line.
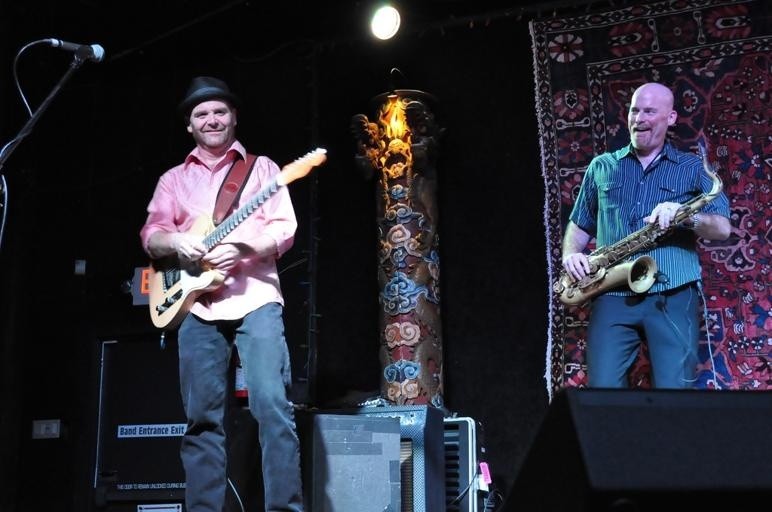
667, 207, 672, 211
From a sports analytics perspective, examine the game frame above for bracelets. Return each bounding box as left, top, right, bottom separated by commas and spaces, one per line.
686, 210, 699, 231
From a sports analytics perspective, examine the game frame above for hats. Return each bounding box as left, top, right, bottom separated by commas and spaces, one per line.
177, 76, 239, 117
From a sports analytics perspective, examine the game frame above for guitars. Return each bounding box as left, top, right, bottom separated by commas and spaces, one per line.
148, 147, 327, 330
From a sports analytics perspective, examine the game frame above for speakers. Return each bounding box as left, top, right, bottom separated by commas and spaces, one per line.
92, 336, 259, 511
504, 388, 772, 512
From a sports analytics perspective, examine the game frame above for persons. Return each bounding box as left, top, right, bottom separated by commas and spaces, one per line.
138, 77, 306, 512
560, 81, 732, 389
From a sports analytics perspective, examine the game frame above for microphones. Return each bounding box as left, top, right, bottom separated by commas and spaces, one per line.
655, 267, 671, 290
48, 35, 108, 65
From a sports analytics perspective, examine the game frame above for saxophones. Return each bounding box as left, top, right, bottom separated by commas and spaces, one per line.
552, 143, 723, 306
